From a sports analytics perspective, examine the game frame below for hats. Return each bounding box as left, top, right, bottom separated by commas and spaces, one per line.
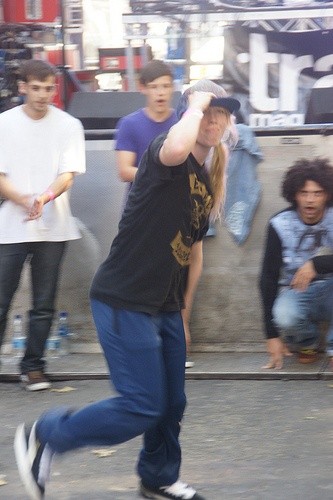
176, 79, 240, 119
143, 60, 173, 83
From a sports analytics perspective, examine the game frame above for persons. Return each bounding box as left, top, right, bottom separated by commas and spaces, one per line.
258, 156, 333, 371
14, 77, 242, 500
0, 60, 89, 394
113, 60, 180, 207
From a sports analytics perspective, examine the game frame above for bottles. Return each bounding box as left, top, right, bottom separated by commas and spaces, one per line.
54, 312, 71, 357
11, 315, 28, 359
45, 313, 61, 358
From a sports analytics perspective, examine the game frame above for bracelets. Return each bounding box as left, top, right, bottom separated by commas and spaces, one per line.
45, 188, 56, 202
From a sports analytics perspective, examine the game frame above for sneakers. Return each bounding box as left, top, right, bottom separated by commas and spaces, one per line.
14, 419, 55, 500
139, 480, 206, 500
185, 356, 194, 367
20, 369, 51, 391
297, 349, 320, 364
